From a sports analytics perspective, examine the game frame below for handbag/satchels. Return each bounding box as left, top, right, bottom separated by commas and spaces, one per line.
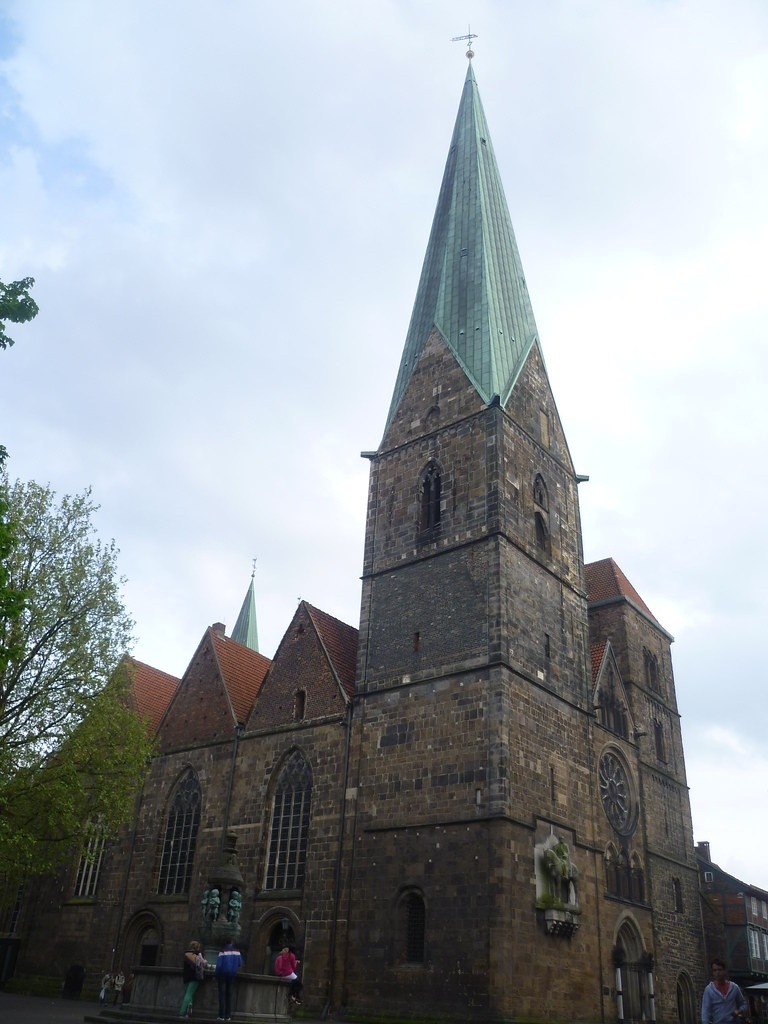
195, 953, 204, 980
113, 975, 119, 987
99, 989, 105, 1000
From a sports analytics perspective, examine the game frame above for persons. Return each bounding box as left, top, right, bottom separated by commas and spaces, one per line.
227, 890, 242, 927
200, 890, 209, 920
98, 970, 135, 1009
701, 958, 748, 1024
179, 940, 216, 1019
274, 944, 305, 1006
214, 935, 244, 1021
207, 889, 220, 923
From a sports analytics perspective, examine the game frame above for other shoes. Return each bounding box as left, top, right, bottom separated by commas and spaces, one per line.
180, 1015, 189, 1019
217, 1017, 224, 1021
226, 1017, 231, 1021
187, 1003, 192, 1018
288, 993, 303, 1008
